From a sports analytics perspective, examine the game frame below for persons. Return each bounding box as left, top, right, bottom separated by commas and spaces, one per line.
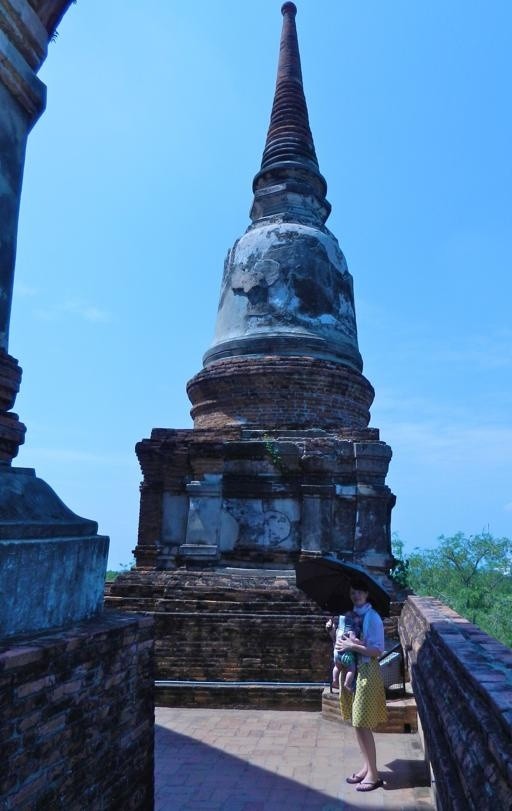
324, 579, 391, 792
331, 610, 366, 693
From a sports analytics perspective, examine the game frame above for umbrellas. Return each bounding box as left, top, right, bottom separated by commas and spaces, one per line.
294, 554, 391, 632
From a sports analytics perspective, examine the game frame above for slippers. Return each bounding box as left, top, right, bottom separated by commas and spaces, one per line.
356, 777, 383, 791
347, 773, 364, 784
